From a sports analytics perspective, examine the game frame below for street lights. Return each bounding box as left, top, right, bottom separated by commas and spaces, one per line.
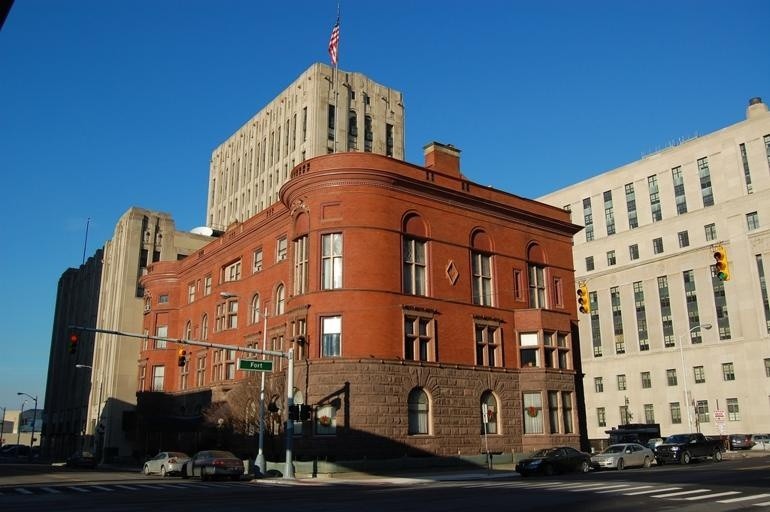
2, 392, 37, 448
679, 323, 712, 434
75, 364, 103, 426
220, 291, 269, 476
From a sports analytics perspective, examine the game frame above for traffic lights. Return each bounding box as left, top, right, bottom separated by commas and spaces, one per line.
713, 246, 730, 281
68, 330, 78, 355
289, 403, 311, 422
577, 283, 590, 314
178, 344, 186, 367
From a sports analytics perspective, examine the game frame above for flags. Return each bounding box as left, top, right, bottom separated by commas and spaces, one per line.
328, 16, 340, 65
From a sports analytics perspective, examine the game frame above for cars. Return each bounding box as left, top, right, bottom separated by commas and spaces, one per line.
515, 434, 769, 475
143, 450, 245, 481
71, 449, 97, 469
0, 445, 42, 456
